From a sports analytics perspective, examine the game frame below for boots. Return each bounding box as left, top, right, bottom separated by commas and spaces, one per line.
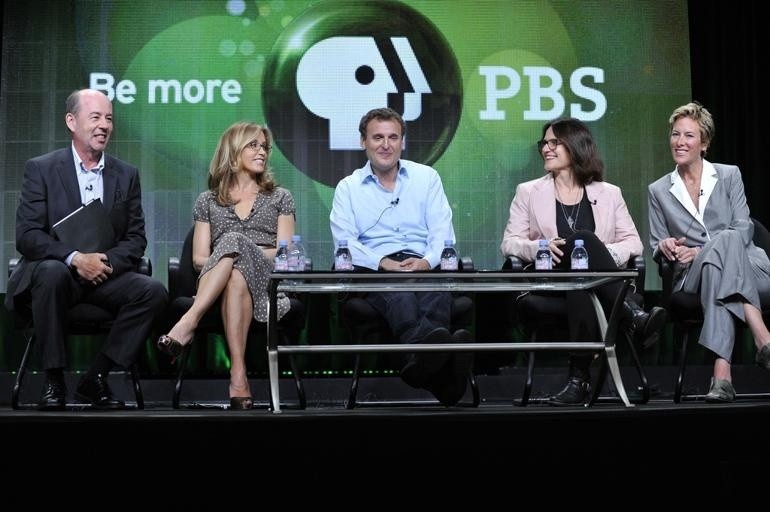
547, 351, 596, 407
623, 297, 668, 350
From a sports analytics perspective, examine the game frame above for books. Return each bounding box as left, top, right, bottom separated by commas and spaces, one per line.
52, 196, 119, 258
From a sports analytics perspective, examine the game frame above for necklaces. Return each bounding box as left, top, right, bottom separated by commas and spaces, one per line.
550, 174, 582, 230
554, 185, 582, 233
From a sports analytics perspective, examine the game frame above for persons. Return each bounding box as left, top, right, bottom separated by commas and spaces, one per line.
11, 86, 172, 414
645, 100, 770, 404
500, 114, 669, 408
156, 119, 301, 413
328, 107, 474, 409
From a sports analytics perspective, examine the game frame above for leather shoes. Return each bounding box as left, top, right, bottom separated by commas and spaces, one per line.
38, 380, 67, 410
73, 380, 126, 410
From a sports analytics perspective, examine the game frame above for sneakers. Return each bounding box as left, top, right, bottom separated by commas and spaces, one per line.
704, 375, 737, 402
753, 342, 770, 375
422, 327, 472, 362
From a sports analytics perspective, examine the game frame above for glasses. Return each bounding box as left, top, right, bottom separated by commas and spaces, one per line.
540, 139, 562, 150
249, 141, 272, 152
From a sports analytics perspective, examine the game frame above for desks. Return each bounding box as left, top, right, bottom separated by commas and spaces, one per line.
268, 271, 638, 412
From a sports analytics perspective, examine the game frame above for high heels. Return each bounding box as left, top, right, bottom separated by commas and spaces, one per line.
157, 334, 194, 366
231, 396, 255, 410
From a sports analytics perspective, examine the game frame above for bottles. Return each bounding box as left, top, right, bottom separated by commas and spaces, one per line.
288, 235, 307, 285
335, 240, 353, 284
441, 241, 460, 282
571, 238, 590, 281
535, 240, 554, 282
273, 239, 289, 288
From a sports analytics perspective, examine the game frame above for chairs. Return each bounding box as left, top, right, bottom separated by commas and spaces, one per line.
506, 253, 649, 407
658, 218, 770, 404
169, 224, 313, 410
8, 255, 152, 410
333, 257, 481, 409
